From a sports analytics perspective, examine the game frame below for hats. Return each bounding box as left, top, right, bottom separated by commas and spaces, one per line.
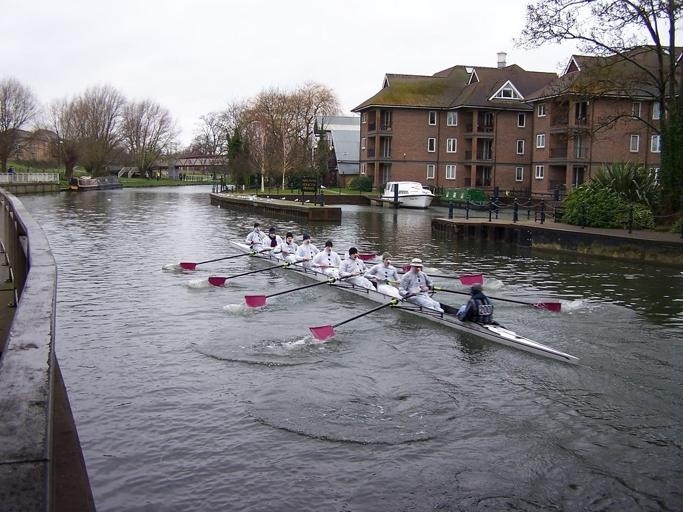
287, 232, 294, 239
303, 232, 311, 241
269, 228, 276, 234
410, 258, 424, 267
382, 252, 392, 260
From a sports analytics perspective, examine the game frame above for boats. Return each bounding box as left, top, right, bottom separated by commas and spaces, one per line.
437, 186, 495, 212
228, 240, 581, 366
67, 175, 123, 191
380, 180, 434, 209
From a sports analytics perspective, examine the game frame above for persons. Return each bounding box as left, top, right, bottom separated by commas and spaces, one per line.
246, 223, 445, 313
457, 283, 494, 326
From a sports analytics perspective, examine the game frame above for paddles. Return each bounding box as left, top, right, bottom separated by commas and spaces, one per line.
363, 261, 411, 272
338, 253, 375, 260
209, 259, 306, 286
397, 271, 483, 284
309, 293, 417, 340
433, 286, 560, 312
180, 249, 272, 269
245, 272, 365, 308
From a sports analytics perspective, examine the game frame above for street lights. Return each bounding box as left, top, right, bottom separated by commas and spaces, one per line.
250, 120, 264, 193
